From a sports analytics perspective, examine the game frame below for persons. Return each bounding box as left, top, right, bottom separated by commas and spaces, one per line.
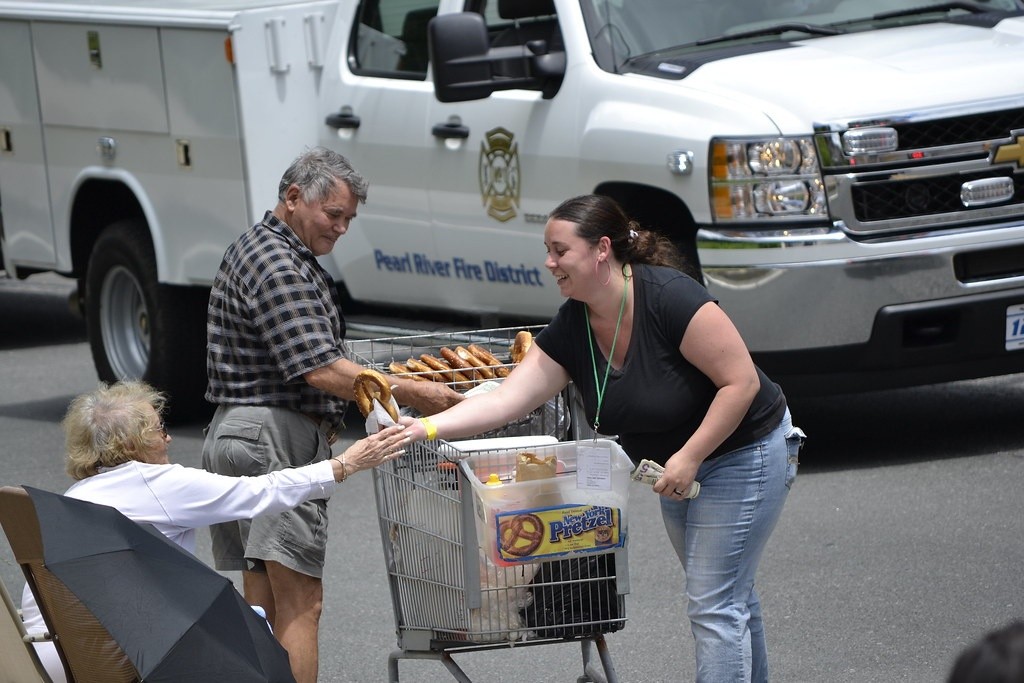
19, 377, 414, 683
395, 191, 806, 683
199, 146, 471, 683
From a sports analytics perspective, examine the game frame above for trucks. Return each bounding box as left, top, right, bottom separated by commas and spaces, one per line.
0, 0, 1023, 427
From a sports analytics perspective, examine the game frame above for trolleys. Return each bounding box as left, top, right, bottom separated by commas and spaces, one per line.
342, 323, 631, 682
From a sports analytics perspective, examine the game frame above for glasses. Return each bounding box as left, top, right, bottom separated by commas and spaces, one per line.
146, 421, 167, 439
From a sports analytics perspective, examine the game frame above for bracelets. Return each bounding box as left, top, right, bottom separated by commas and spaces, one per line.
418, 416, 437, 441
331, 457, 349, 484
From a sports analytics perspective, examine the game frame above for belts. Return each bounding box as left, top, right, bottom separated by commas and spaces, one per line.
300, 410, 337, 446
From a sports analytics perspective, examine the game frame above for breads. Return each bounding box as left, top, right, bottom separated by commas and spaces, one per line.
389, 330, 532, 389
352, 369, 398, 425
497, 513, 543, 557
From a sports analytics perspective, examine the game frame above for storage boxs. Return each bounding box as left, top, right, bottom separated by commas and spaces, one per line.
460, 438, 634, 566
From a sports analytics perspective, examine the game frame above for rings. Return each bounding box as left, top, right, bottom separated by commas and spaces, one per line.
674, 489, 682, 496
